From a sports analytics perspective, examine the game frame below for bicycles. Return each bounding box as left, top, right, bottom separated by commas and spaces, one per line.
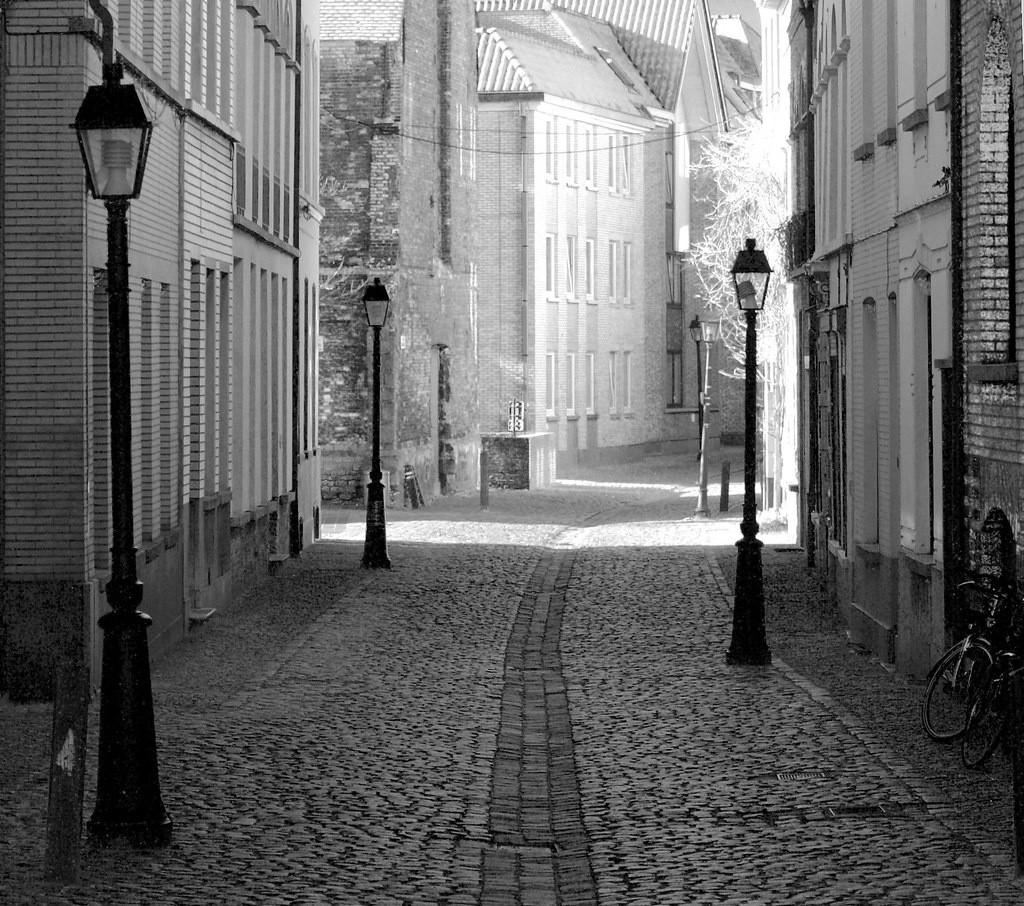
922, 581, 1024, 769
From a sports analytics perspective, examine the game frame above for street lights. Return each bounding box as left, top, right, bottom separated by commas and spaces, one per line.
359, 277, 392, 571
694, 318, 720, 516
725, 238, 773, 666
68, 61, 174, 852
687, 317, 702, 460
